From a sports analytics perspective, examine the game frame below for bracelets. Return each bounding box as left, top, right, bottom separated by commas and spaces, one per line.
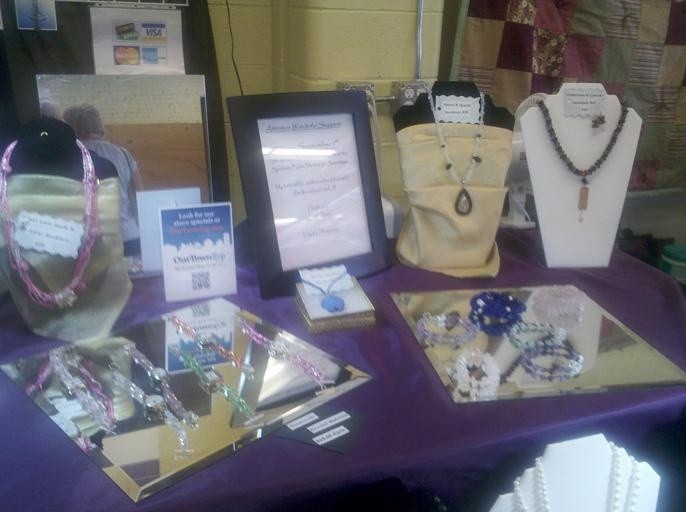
467, 310, 522, 336
465, 291, 527, 317
413, 309, 478, 346
505, 321, 568, 347
451, 347, 500, 395
531, 283, 587, 310
519, 346, 583, 379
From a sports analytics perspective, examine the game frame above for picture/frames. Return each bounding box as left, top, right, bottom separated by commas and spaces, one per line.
225, 90, 393, 297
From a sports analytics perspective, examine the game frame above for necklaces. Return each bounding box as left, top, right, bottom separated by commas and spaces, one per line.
23, 351, 120, 425
298, 270, 351, 313
534, 440, 625, 512
513, 453, 640, 512
537, 95, 629, 224
0, 137, 99, 310
421, 86, 487, 217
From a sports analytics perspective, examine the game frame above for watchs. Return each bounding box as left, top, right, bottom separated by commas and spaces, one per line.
239, 318, 325, 382
101, 353, 187, 454
161, 313, 255, 376
165, 341, 256, 420
43, 348, 117, 435
121, 340, 199, 428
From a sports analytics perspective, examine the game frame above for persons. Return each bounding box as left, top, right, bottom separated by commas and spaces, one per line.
60, 101, 146, 260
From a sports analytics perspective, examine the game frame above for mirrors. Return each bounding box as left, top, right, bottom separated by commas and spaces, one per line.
34, 69, 223, 284
